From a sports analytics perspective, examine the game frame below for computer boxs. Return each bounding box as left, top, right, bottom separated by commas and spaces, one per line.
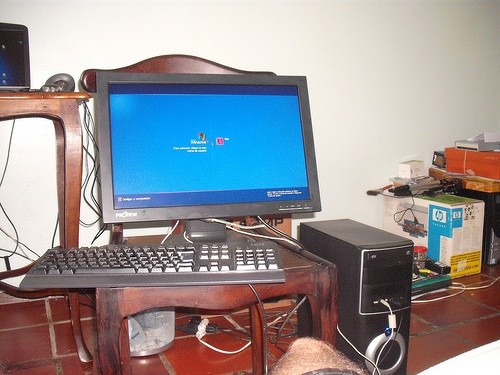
298, 218, 416, 374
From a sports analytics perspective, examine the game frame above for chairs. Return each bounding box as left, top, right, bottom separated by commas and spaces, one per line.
79, 54, 338, 375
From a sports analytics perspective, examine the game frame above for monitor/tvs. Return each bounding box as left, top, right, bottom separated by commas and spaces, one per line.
94, 70, 321, 248
0, 22, 31, 92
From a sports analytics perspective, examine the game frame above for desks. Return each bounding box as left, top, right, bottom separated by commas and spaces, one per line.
429, 165, 500, 265
0, 92, 94, 363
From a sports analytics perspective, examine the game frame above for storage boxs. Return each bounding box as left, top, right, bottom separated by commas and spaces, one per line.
445, 147, 500, 181
454, 140, 500, 151
383, 193, 485, 279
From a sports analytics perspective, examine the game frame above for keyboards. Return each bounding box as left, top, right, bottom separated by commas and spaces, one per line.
18, 240, 287, 287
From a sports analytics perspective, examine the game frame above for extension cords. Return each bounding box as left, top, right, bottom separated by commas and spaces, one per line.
183, 323, 219, 333
